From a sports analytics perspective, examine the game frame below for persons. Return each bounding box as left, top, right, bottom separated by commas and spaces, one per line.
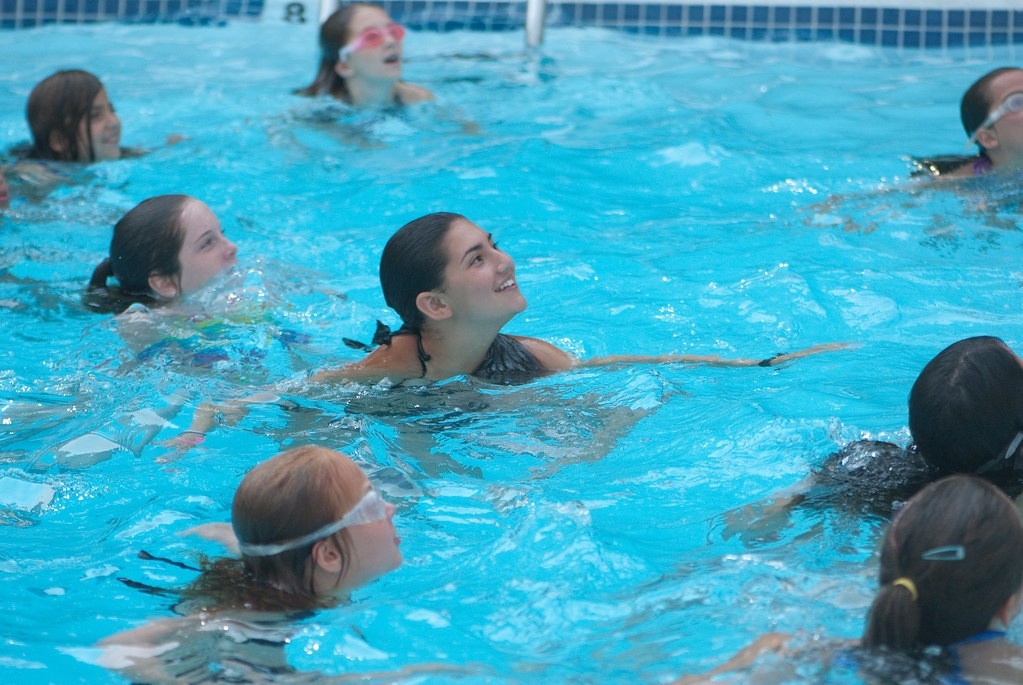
9, 70, 184, 191
665, 474, 1023, 685
260, 4, 483, 167
95, 446, 474, 684
0, 174, 10, 211
88, 194, 348, 383
802, 67, 1022, 236
628, 336, 1023, 620
149, 212, 858, 467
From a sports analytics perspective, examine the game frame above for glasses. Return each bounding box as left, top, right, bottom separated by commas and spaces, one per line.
969, 90, 1021, 142
235, 485, 386, 558
338, 24, 406, 61
